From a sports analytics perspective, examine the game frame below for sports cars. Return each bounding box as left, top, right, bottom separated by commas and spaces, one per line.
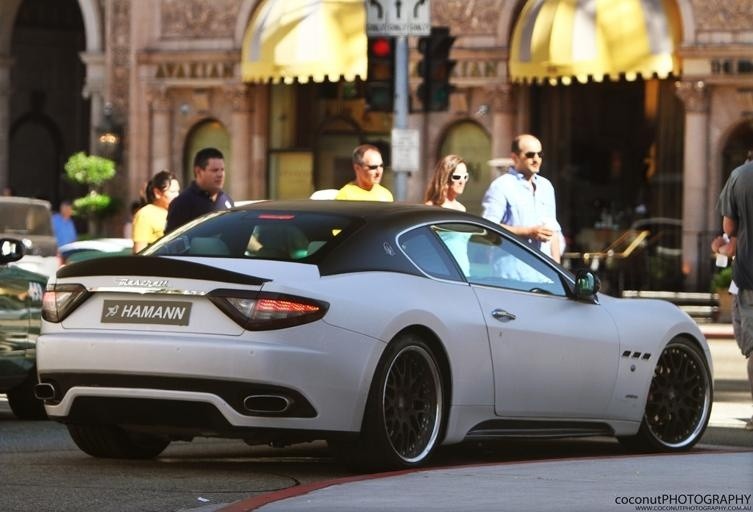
35, 200, 714, 465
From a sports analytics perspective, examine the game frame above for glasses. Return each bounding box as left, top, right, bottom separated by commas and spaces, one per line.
525, 152, 544, 158
368, 164, 382, 169
450, 173, 469, 182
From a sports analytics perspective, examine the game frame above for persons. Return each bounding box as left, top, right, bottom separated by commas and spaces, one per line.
132, 170, 182, 254
711, 151, 753, 431
334, 142, 393, 202
479, 132, 567, 283
122, 200, 144, 239
163, 146, 236, 237
424, 154, 469, 277
2, 185, 16, 196
51, 200, 78, 246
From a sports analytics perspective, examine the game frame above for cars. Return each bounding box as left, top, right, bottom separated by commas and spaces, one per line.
619, 210, 680, 277
0, 191, 332, 417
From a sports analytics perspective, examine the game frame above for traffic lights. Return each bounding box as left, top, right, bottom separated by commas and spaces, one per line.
426, 24, 456, 114
363, 31, 396, 112
415, 33, 427, 106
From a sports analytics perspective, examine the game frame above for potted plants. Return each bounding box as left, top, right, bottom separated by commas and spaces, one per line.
708, 266, 733, 324
